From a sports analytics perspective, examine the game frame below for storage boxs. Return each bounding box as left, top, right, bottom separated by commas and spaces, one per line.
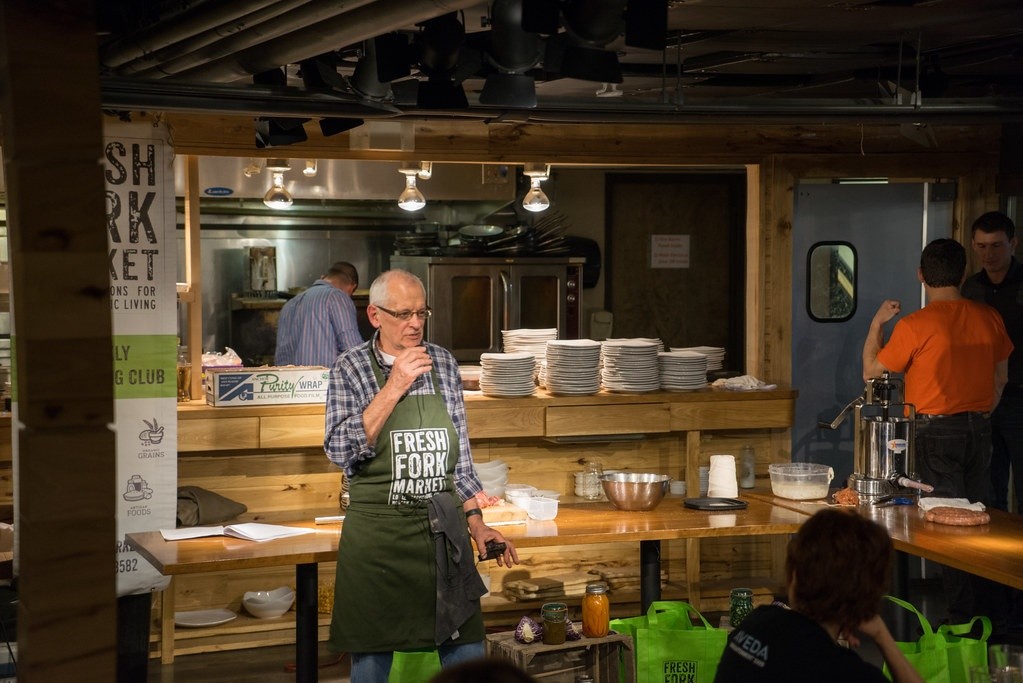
482, 623, 637, 683
204, 366, 331, 408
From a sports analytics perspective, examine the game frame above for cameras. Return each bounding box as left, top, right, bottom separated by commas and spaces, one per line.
477, 542, 507, 562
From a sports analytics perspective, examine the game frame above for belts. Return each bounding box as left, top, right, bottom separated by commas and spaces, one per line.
916, 411, 990, 421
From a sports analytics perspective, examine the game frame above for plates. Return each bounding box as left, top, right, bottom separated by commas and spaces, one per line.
669, 465, 710, 496
174, 608, 237, 628
479, 329, 726, 396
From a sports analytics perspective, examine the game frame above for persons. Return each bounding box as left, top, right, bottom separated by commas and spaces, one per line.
863, 235, 1015, 643
274, 260, 365, 369
325, 266, 519, 683
427, 655, 540, 683
712, 509, 925, 683
962, 210, 1023, 518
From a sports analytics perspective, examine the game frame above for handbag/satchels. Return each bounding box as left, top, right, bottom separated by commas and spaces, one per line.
881, 595, 950, 683
937, 615, 992, 683
609, 601, 728, 683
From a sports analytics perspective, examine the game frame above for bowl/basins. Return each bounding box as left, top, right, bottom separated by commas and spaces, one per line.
599, 471, 671, 510
458, 366, 482, 390
243, 586, 296, 618
464, 459, 561, 521
768, 462, 834, 501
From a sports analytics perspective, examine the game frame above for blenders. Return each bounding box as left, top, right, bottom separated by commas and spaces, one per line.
831, 370, 935, 503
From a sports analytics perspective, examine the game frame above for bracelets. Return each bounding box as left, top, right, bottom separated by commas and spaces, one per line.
466, 507, 482, 518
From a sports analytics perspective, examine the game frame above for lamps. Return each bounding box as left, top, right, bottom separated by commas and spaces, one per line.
397, 162, 427, 213
255, 0, 675, 149
261, 159, 295, 210
522, 163, 550, 214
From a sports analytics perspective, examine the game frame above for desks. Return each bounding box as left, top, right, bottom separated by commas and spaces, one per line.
124, 488, 811, 683
742, 476, 1023, 641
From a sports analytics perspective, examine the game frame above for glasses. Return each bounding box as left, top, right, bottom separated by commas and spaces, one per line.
373, 304, 432, 321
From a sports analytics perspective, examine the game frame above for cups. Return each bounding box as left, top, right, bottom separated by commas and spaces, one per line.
969, 645, 1023, 683
572, 455, 618, 499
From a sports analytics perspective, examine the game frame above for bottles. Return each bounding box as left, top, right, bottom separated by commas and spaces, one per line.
582, 584, 610, 638
739, 443, 757, 488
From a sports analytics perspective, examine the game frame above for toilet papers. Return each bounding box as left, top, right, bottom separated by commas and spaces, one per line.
706, 455, 739, 498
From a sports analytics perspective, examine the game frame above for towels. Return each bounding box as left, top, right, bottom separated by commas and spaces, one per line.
712, 375, 778, 390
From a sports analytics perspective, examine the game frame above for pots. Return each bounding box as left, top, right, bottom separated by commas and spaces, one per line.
457, 210, 575, 255
